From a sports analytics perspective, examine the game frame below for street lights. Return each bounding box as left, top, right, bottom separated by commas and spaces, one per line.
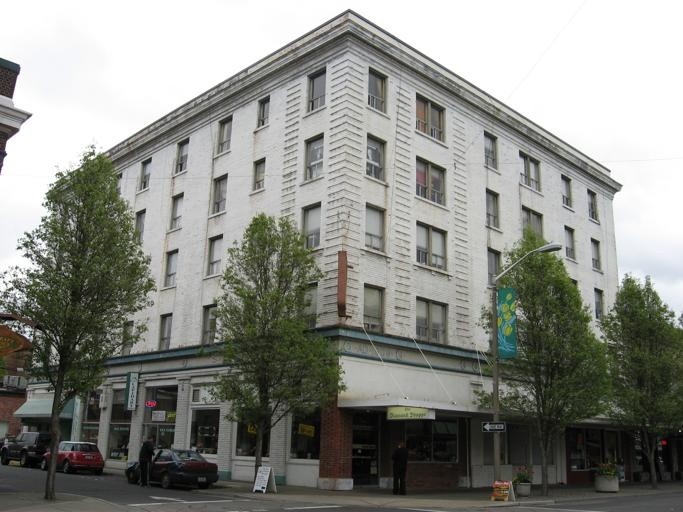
490, 241, 562, 482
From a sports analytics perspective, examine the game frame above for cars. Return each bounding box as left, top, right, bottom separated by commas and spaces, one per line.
39, 440, 105, 475
0, 431, 57, 468
124, 447, 219, 491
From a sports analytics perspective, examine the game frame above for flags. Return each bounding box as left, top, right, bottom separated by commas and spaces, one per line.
495, 284, 519, 361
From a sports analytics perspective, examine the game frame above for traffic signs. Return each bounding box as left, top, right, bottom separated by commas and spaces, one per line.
482, 422, 506, 432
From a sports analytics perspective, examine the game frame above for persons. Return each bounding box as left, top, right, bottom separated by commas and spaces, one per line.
389, 439, 410, 496
138, 435, 154, 488
